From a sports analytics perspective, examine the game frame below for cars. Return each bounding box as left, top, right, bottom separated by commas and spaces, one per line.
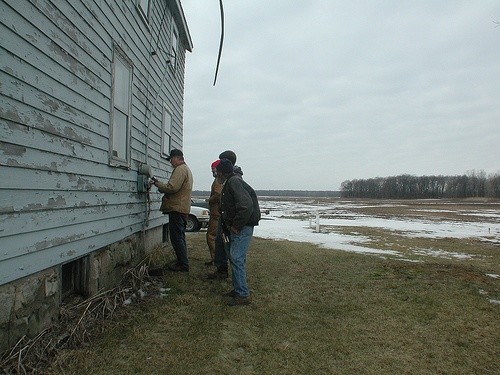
186, 200, 210, 232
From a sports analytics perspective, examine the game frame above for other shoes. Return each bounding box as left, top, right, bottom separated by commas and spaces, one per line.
205, 259, 216, 264
168, 262, 190, 273
211, 272, 228, 280
226, 289, 251, 305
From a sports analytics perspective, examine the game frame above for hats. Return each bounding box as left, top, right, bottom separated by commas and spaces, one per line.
216, 159, 233, 173
212, 160, 221, 166
166, 148, 183, 159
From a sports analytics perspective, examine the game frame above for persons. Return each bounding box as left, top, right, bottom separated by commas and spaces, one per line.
149, 149, 193, 272
204, 151, 261, 303
